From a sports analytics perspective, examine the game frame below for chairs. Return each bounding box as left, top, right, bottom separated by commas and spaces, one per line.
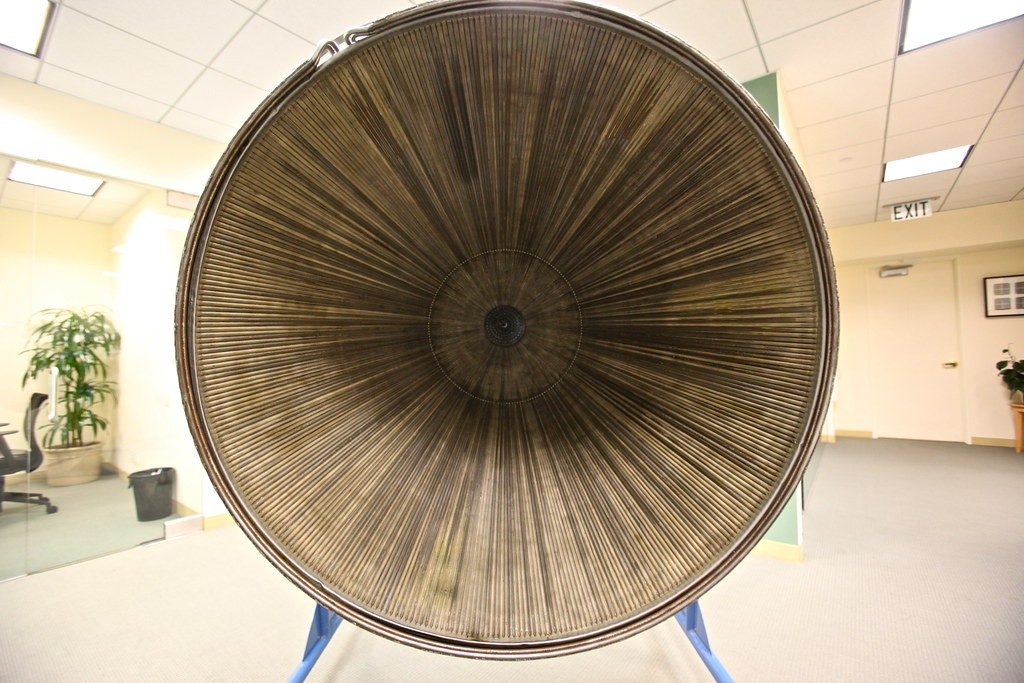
0, 393, 57, 514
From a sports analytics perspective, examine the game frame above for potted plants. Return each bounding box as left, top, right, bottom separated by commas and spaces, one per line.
19, 310, 120, 487
997, 349, 1024, 454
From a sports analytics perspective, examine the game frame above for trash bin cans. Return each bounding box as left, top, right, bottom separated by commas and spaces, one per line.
126, 467, 175, 522
